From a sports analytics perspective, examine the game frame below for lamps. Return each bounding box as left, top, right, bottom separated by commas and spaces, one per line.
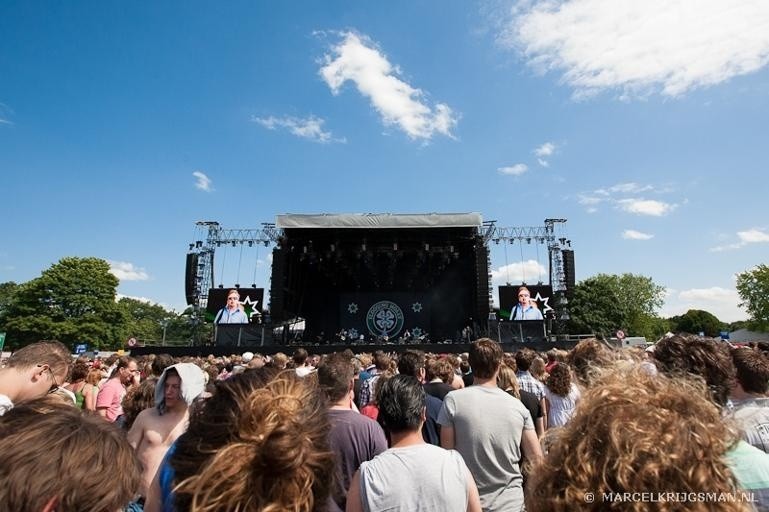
495, 237, 572, 248
188, 239, 271, 249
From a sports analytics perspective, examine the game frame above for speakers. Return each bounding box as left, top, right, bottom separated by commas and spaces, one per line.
186, 254, 198, 304
562, 250, 575, 302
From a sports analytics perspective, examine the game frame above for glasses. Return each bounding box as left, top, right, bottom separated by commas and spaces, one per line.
38, 363, 59, 395
519, 294, 529, 297
228, 297, 239, 300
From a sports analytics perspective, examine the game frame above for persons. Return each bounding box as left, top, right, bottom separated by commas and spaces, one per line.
509, 286, 543, 321
214, 289, 249, 325
